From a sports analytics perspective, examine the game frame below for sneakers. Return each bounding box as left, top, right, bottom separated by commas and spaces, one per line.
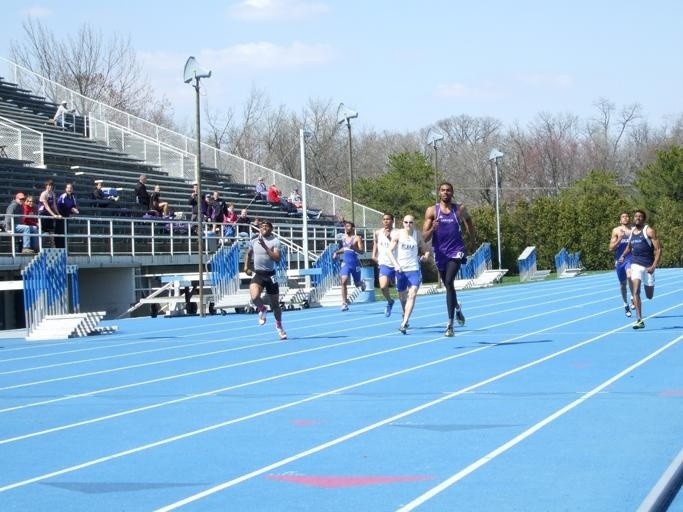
399, 325, 406, 334
258, 307, 267, 325
625, 297, 644, 329
456, 306, 465, 327
386, 302, 392, 317
276, 325, 286, 340
445, 325, 454, 336
341, 300, 351, 312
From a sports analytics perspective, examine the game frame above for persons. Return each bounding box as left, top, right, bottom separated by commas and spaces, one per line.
386, 214, 431, 334
372, 213, 400, 317
4, 179, 80, 252
52, 100, 75, 128
244, 221, 288, 340
609, 211, 635, 317
91, 182, 119, 208
133, 174, 323, 240
618, 209, 662, 330
422, 182, 475, 336
331, 222, 366, 312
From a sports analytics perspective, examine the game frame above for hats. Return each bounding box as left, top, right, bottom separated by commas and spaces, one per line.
16, 193, 25, 200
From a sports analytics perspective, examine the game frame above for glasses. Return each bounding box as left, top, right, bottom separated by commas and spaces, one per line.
404, 221, 413, 225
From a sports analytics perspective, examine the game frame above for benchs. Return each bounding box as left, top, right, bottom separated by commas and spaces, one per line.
0, 75, 378, 257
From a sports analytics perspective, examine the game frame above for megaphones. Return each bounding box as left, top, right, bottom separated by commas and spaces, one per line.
489, 148, 503, 160
336, 102, 358, 124
426, 128, 444, 144
184, 56, 211, 83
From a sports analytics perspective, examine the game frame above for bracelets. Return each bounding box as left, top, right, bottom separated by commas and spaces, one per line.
620, 254, 626, 258
